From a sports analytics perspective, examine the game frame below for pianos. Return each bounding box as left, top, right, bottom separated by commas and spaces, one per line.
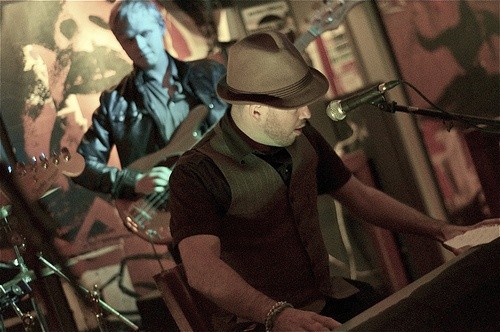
322, 215, 500, 332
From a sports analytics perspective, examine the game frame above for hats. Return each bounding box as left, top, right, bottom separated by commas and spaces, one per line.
217, 30, 330, 110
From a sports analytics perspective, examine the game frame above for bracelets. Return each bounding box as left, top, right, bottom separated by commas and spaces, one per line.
264, 301, 293, 332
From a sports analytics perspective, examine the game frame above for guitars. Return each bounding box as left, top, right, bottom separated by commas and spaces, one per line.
115, 0, 361, 246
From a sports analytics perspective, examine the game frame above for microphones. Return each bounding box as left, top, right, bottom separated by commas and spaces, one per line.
326, 81, 400, 122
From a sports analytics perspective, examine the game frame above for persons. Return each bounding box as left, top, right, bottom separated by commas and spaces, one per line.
167, 29, 500, 332
71, 0, 227, 266
